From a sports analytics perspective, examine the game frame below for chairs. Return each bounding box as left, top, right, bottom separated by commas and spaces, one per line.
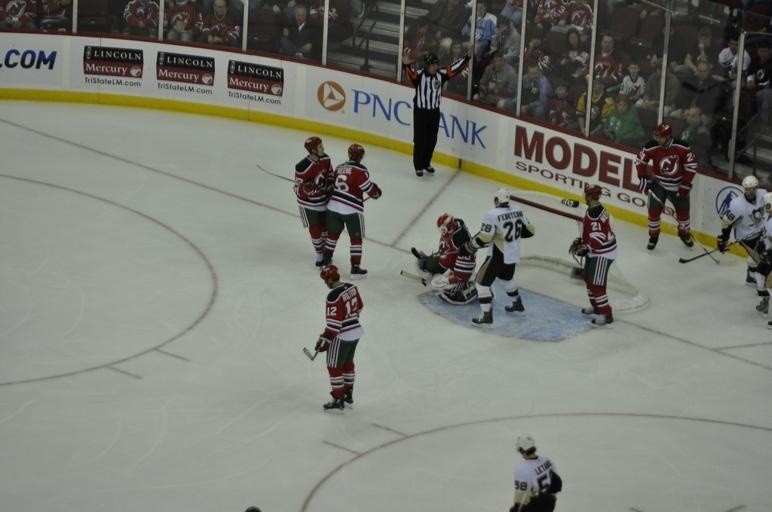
191, 0, 380, 61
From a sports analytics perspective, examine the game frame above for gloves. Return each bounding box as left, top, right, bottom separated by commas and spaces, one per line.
677, 181, 693, 199
639, 174, 652, 195
314, 334, 332, 351
569, 237, 591, 258
716, 227, 731, 253
457, 242, 476, 256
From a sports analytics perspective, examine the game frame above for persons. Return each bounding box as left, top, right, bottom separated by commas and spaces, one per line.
318, 144, 381, 279
464, 188, 535, 329
569, 185, 618, 329
740, 193, 772, 326
395, 1, 772, 189
314, 264, 363, 410
508, 434, 557, 511
418, 214, 477, 305
715, 175, 771, 298
1, 1, 371, 64
292, 137, 334, 267
635, 123, 698, 250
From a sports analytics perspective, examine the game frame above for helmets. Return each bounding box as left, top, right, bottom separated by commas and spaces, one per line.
319, 265, 339, 280
424, 51, 440, 65
493, 188, 510, 203
742, 174, 759, 190
436, 212, 458, 234
348, 143, 364, 160
655, 121, 673, 136
584, 184, 601, 200
305, 136, 322, 154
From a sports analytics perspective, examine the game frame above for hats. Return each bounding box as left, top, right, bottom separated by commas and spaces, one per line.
515, 431, 535, 451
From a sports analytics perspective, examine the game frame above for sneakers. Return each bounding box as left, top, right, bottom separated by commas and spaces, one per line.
646, 235, 659, 250
746, 265, 769, 296
415, 163, 435, 176
677, 229, 693, 246
314, 260, 331, 267
350, 265, 367, 275
471, 307, 494, 324
505, 295, 524, 312
592, 316, 613, 324
582, 306, 600, 316
323, 390, 353, 408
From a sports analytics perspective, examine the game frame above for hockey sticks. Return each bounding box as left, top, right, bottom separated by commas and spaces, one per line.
254, 159, 307, 186
647, 187, 722, 265
302, 347, 318, 361
675, 236, 745, 263
